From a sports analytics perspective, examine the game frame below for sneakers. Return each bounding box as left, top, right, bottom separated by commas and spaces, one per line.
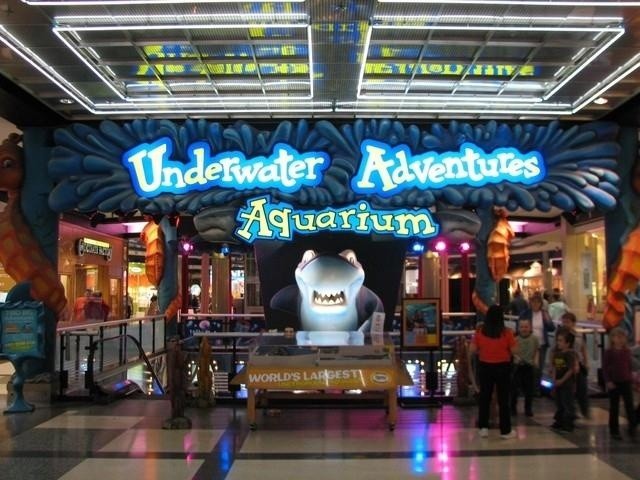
478, 428, 488, 437
501, 429, 517, 439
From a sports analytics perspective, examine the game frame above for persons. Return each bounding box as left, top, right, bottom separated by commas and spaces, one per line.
604, 327, 640, 444
57, 287, 160, 325
468, 284, 590, 438
